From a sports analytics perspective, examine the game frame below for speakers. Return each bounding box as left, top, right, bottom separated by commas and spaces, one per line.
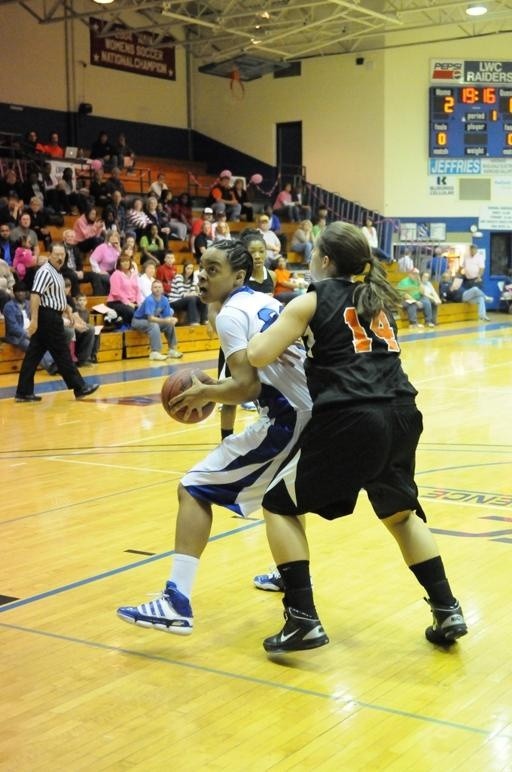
80, 103, 92, 114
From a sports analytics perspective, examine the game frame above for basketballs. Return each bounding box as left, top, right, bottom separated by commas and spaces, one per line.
161, 368, 217, 424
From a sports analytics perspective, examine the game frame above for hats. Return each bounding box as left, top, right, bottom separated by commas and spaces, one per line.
203, 170, 270, 222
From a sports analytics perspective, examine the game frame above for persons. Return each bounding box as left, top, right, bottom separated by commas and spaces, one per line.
249, 222, 466, 654
13, 242, 99, 403
0, 123, 495, 377
117, 241, 314, 637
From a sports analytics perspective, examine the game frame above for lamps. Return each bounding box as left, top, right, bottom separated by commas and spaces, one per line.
464, 0, 487, 16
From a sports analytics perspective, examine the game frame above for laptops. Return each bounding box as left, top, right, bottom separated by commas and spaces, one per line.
64, 146, 79, 159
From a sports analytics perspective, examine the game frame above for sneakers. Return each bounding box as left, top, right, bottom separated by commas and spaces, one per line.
254, 567, 313, 593
16, 392, 41, 402
74, 383, 98, 398
168, 350, 182, 358
150, 352, 167, 360
118, 580, 194, 634
47, 362, 58, 374
264, 599, 329, 650
408, 297, 494, 328
423, 595, 467, 643
